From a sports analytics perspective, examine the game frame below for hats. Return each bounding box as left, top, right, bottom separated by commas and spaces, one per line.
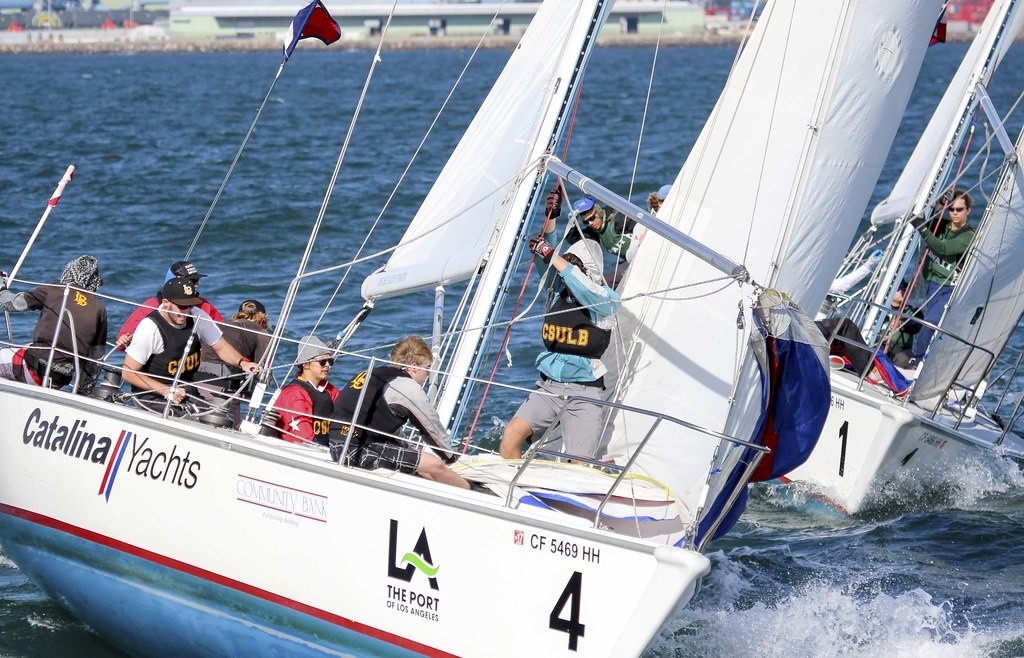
60, 254, 102, 291
162, 278, 203, 306
900, 278, 908, 286
170, 262, 207, 280
297, 336, 331, 363
239, 300, 265, 315
572, 197, 597, 214
658, 184, 672, 200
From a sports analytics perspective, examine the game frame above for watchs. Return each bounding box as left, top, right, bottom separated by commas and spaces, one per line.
237, 357, 249, 369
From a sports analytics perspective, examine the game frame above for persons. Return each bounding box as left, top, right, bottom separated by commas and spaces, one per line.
500, 182, 620, 466
567, 197, 637, 288
0, 254, 108, 393
829, 249, 882, 293
330, 336, 474, 489
626, 184, 672, 263
866, 277, 923, 369
121, 279, 263, 416
910, 188, 974, 371
195, 300, 271, 428
274, 336, 340, 447
116, 261, 225, 350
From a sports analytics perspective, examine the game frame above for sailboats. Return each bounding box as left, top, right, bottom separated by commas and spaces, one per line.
0, 1, 951, 658
778, 1, 1024, 518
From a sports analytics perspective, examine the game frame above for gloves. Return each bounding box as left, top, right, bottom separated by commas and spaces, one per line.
910, 212, 927, 232
545, 185, 562, 220
867, 249, 882, 266
528, 234, 554, 264
0, 271, 8, 292
939, 189, 953, 205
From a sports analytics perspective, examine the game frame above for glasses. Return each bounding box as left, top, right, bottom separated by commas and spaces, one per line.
583, 211, 597, 225
897, 287, 906, 291
319, 358, 334, 366
191, 279, 199, 285
401, 364, 431, 374
162, 297, 194, 310
948, 207, 967, 212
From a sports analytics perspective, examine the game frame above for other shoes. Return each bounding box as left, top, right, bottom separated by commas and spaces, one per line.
870, 369, 883, 382
